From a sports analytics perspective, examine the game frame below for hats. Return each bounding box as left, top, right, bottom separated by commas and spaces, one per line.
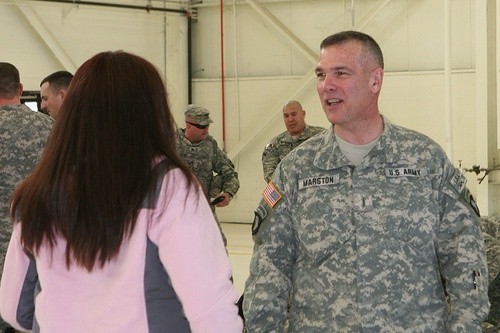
184, 104, 214, 127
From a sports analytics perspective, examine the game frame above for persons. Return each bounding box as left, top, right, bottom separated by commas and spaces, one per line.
242, 30, 491, 333
40, 70, 74, 121
261, 100, 327, 184
0, 50, 241, 333
0, 62, 57, 333
173, 104, 240, 212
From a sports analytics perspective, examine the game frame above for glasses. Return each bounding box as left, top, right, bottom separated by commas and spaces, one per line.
184, 121, 209, 129
